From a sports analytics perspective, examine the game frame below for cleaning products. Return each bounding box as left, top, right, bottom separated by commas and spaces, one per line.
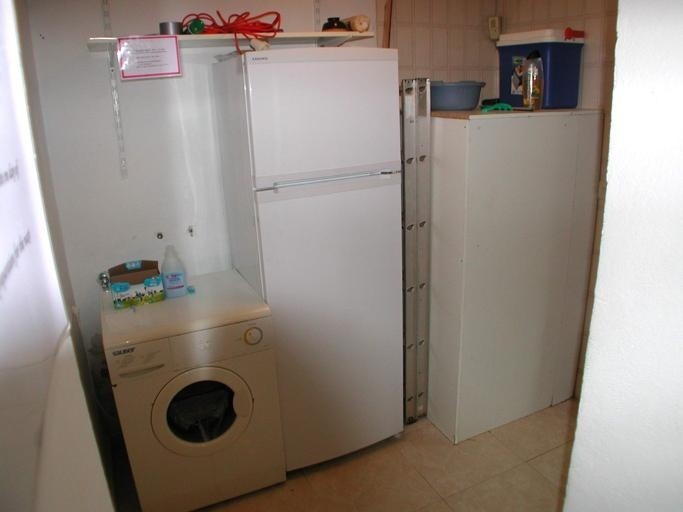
161, 245, 186, 297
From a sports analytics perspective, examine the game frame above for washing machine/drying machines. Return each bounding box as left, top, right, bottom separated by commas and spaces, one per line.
100, 270, 286, 512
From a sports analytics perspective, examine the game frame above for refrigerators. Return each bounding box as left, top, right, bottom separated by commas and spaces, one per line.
212, 48, 405, 476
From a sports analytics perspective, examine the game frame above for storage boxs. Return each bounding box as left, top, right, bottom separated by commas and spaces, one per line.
495, 29, 585, 111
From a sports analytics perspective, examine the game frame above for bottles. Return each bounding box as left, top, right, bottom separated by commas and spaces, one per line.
522, 49, 545, 111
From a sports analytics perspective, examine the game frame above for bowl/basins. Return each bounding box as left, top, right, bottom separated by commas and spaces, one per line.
322, 18, 349, 32
430, 82, 487, 111
